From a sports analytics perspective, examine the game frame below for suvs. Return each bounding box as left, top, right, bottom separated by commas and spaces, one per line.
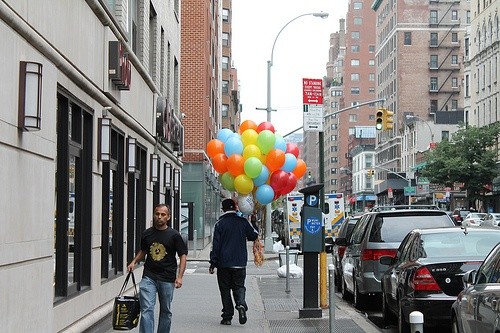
334, 204, 457, 311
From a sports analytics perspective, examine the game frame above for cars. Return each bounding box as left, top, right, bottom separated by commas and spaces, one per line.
454, 209, 475, 226
325, 215, 360, 294
378, 224, 500, 333
449, 240, 499, 333
478, 212, 500, 226
461, 213, 488, 227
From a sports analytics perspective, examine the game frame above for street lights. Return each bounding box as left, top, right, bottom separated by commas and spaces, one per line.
306, 167, 312, 179
406, 114, 436, 205
340, 168, 357, 211
256, 10, 331, 254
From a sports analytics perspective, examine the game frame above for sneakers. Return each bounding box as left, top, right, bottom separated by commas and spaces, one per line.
221, 317, 232, 325
239, 305, 247, 324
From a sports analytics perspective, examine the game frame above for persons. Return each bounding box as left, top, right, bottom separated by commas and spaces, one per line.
486, 203, 495, 213
128, 204, 188, 333
208, 199, 259, 325
480, 206, 485, 213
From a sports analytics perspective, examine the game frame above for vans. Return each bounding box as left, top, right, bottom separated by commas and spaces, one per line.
452, 207, 476, 223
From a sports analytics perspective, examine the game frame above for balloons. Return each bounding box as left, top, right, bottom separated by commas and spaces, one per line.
207, 120, 306, 206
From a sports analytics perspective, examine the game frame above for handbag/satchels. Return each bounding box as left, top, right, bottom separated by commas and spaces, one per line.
112, 272, 140, 330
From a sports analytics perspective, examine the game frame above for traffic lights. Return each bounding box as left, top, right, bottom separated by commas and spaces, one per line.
371, 170, 375, 175
384, 108, 394, 132
367, 169, 371, 177
374, 108, 385, 132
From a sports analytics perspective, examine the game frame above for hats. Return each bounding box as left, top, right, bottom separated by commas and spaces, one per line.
221, 199, 235, 209
